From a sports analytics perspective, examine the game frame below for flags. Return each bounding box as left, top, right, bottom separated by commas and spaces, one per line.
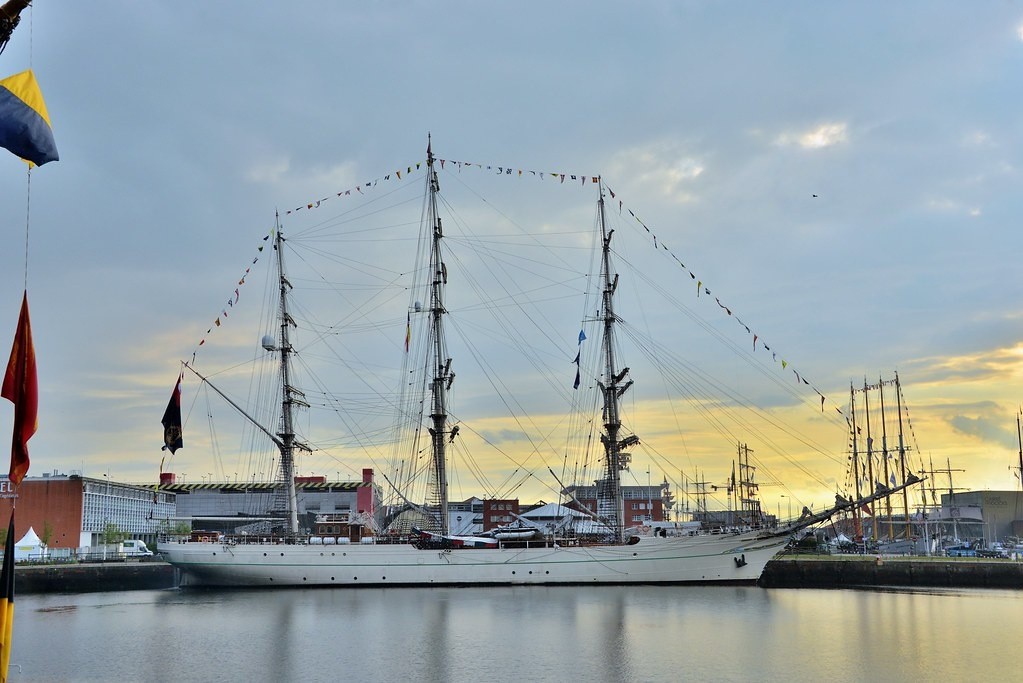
572, 330, 586, 390
858, 426, 924, 526
146, 376, 183, 520
0, 69, 60, 170
404, 311, 412, 352
0, 508, 14, 683
0, 290, 39, 484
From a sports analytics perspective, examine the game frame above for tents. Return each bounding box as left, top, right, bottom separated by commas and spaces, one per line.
15, 526, 48, 562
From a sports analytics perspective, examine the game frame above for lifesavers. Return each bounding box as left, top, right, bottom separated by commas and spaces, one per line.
636, 525, 643, 532
202, 536, 209, 542
643, 526, 650, 532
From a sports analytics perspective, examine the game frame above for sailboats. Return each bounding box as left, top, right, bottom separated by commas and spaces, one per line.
140, 133, 931, 586
642, 365, 1022, 560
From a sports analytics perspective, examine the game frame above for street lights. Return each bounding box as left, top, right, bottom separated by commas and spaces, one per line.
337, 472, 340, 483
251, 475, 255, 482
201, 476, 207, 484
226, 475, 230, 484
103, 468, 115, 561
260, 472, 264, 482
348, 474, 351, 483
208, 472, 213, 484
182, 473, 187, 484
311, 471, 314, 477
235, 473, 238, 483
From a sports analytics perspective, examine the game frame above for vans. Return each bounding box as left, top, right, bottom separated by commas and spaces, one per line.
123, 540, 154, 558
